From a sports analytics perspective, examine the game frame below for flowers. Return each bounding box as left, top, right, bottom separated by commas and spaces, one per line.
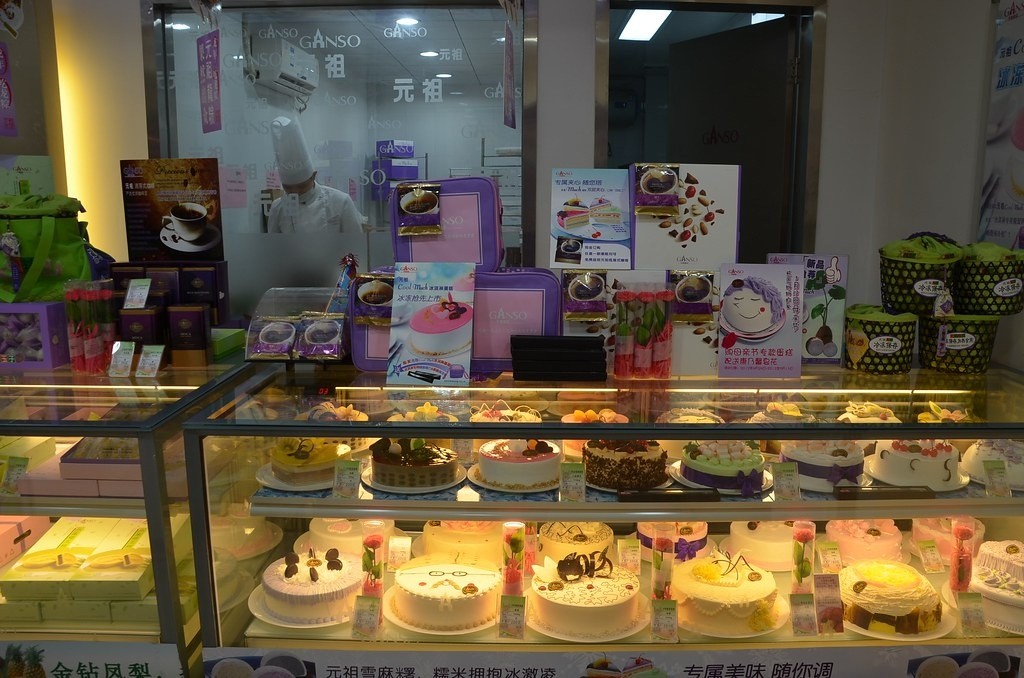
66, 289, 112, 337
362, 525, 973, 627
612, 290, 675, 379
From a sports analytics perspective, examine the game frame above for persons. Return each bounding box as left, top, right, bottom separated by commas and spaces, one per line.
268, 165, 366, 262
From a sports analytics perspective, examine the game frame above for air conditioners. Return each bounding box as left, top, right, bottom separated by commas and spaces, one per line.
252, 35, 319, 98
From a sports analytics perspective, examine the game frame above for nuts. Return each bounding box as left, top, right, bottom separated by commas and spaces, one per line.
660, 196, 710, 236
694, 286, 719, 334
586, 285, 617, 334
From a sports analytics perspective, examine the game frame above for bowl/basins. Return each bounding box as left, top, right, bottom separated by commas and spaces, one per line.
357, 281, 394, 307
304, 320, 342, 344
259, 321, 295, 347
567, 274, 605, 301
675, 276, 713, 303
561, 240, 582, 254
400, 191, 438, 215
639, 167, 678, 195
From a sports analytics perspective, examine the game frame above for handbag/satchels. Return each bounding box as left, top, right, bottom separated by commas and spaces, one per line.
387, 175, 505, 272
350, 266, 395, 372
0, 192, 116, 303
470, 267, 563, 373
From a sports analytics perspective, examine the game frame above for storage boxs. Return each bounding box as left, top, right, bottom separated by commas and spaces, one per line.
371, 140, 419, 201
211, 328, 245, 359
0, 406, 236, 624
107, 262, 230, 367
510, 334, 607, 382
0, 301, 69, 368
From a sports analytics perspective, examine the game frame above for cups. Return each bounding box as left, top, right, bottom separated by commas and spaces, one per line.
161, 201, 208, 243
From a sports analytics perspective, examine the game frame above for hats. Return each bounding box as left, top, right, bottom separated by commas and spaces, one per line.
271, 115, 314, 186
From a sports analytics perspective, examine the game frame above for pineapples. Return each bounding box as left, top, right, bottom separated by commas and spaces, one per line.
4, 644, 46, 678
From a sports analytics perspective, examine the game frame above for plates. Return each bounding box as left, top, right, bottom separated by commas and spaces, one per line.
719, 298, 787, 343
160, 223, 221, 253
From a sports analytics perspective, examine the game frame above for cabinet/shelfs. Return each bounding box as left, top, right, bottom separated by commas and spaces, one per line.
1, 349, 268, 677
180, 363, 1024, 650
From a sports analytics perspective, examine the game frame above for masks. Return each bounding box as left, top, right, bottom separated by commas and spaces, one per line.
284, 188, 318, 204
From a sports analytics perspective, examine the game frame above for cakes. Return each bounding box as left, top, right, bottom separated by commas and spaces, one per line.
556, 194, 622, 230
584, 650, 654, 678
407, 291, 472, 356
267, 401, 1024, 492
723, 276, 784, 333
260, 515, 1024, 638
